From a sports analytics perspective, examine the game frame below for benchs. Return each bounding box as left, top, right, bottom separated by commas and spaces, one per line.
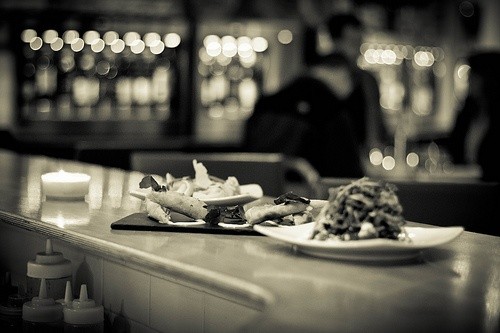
133, 150, 326, 196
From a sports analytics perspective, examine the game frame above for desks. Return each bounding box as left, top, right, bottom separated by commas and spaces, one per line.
370, 169, 481, 189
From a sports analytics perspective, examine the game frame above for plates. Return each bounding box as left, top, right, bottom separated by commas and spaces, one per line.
252, 218, 464, 261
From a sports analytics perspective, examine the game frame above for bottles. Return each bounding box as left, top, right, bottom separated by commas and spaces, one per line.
24, 237, 73, 302
63, 284, 105, 333
0, 271, 32, 333
56, 281, 76, 307
22, 278, 63, 333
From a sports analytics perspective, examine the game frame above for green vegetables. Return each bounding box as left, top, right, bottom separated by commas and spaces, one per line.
312, 175, 401, 244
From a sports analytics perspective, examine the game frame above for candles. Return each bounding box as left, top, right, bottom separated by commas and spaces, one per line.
42, 168, 90, 197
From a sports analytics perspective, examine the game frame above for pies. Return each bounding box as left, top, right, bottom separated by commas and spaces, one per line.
244, 199, 308, 224
143, 191, 209, 224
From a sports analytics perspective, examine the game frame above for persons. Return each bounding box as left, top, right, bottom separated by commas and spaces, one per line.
287, 13, 385, 187
